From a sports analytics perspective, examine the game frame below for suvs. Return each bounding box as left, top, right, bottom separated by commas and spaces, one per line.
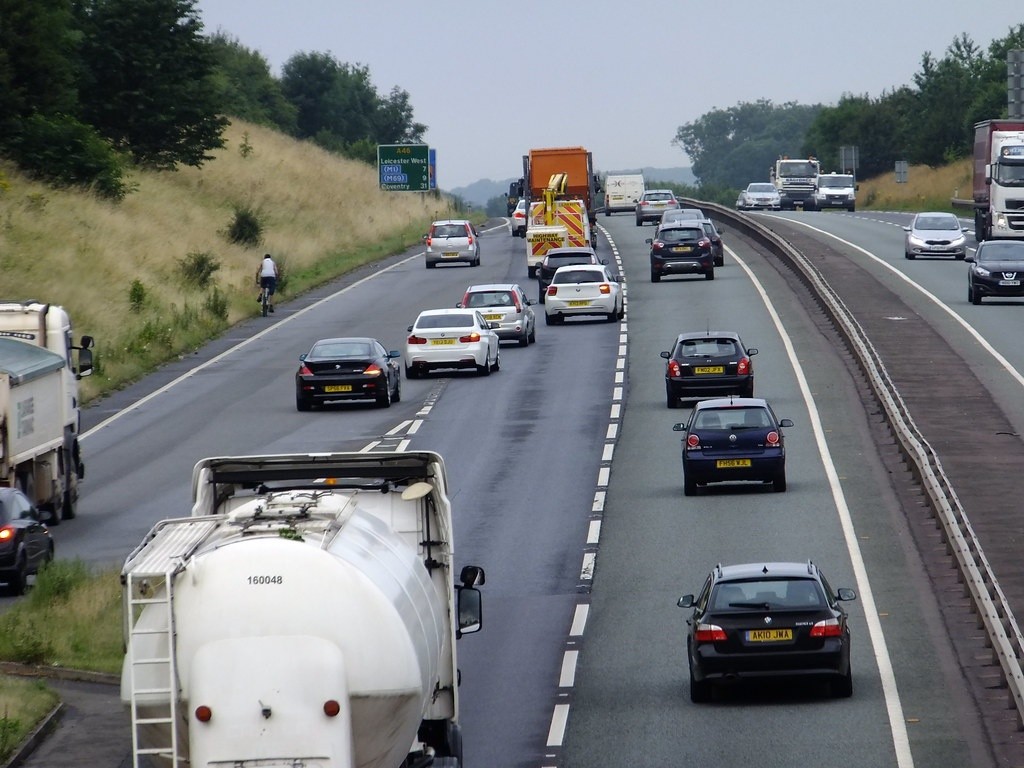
645, 226, 715, 283
535, 247, 610, 304
652, 209, 724, 267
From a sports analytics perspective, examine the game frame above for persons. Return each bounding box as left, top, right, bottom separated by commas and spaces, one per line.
256, 253, 278, 312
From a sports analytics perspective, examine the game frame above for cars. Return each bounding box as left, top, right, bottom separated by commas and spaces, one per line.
904, 212, 970, 260
295, 337, 402, 408
456, 283, 538, 347
676, 555, 858, 705
735, 183, 782, 211
403, 308, 500, 380
511, 200, 527, 237
544, 264, 625, 327
964, 239, 1024, 305
633, 189, 681, 227
672, 398, 795, 496
0, 487, 56, 596
661, 331, 758, 409
421, 219, 480, 268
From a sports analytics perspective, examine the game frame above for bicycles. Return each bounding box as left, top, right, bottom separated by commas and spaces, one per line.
258, 281, 272, 317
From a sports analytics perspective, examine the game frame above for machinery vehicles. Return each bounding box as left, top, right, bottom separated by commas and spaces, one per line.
525, 172, 592, 280
119, 450, 486, 768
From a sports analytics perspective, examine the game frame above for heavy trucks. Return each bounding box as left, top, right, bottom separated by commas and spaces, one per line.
0, 297, 95, 530
504, 145, 601, 250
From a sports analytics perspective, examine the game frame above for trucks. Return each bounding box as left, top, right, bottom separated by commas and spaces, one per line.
817, 170, 859, 212
972, 118, 1024, 242
770, 154, 821, 210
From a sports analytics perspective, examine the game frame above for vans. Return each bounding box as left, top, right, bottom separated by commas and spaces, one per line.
602, 174, 645, 216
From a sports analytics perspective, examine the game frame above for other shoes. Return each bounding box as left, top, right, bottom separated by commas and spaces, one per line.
257, 296, 262, 302
269, 308, 274, 313
